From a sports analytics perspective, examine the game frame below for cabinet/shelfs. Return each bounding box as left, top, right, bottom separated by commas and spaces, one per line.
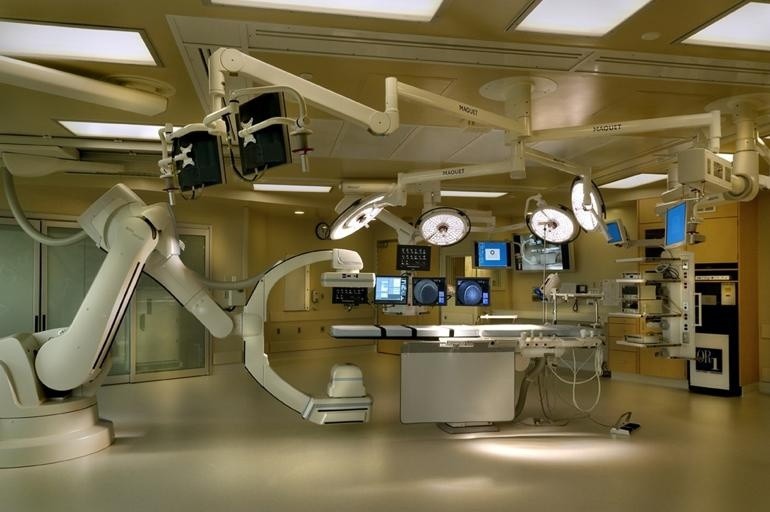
637, 195, 688, 380
608, 316, 640, 375
615, 256, 681, 348
687, 193, 759, 264
269, 311, 374, 353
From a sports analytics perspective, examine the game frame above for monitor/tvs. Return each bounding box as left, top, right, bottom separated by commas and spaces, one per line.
454, 277, 492, 307
603, 217, 627, 246
171, 130, 228, 192
412, 277, 447, 306
374, 275, 408, 305
510, 232, 576, 273
396, 244, 432, 271
471, 239, 515, 270
664, 199, 689, 250
331, 286, 369, 305
234, 91, 293, 177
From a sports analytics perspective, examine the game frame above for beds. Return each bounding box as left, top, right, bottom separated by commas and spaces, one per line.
328, 323, 606, 341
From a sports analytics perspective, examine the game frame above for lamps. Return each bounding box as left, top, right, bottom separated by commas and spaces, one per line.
526, 202, 581, 245
570, 175, 606, 233
414, 205, 471, 248
330, 192, 385, 241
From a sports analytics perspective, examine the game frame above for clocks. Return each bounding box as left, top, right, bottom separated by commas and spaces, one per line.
315, 222, 330, 240
